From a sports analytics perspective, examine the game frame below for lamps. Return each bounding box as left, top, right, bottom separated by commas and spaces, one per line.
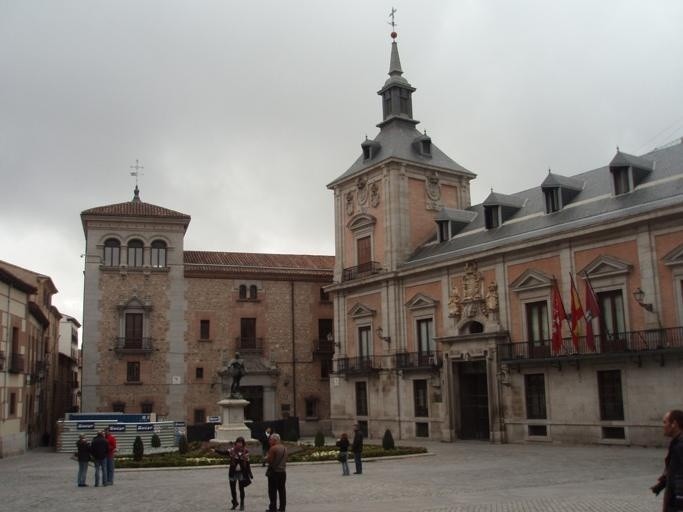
632, 287, 653, 312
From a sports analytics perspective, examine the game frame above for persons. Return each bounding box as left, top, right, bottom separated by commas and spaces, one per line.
335, 433, 352, 475
76, 431, 91, 487
448, 287, 462, 316
485, 282, 498, 310
89, 429, 110, 487
208, 436, 250, 512
648, 408, 682, 511
350, 423, 364, 474
223, 350, 248, 400
260, 424, 274, 468
101, 426, 116, 485
264, 433, 288, 512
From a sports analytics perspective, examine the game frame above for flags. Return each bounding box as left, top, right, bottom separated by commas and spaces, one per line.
564, 265, 584, 354
550, 275, 567, 354
580, 269, 601, 355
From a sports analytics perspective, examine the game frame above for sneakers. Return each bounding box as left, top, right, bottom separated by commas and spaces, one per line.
266, 509, 286, 512
231, 502, 244, 511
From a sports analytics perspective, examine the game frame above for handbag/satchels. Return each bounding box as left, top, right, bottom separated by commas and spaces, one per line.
265, 465, 271, 476
240, 473, 251, 486
336, 454, 347, 462
